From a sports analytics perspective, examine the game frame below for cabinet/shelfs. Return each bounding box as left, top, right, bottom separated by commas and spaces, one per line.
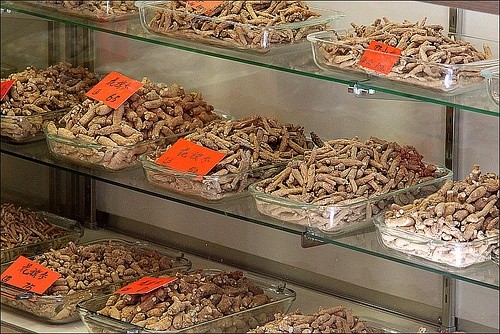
0, 1, 500, 334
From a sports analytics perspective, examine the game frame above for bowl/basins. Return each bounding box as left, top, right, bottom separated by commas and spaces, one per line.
43, 109, 235, 174
76, 269, 298, 334
137, 151, 280, 206
247, 161, 454, 235
26, 1, 140, 23
1, 108, 67, 145
0, 238, 193, 326
374, 197, 499, 272
0, 212, 84, 266
306, 24, 500, 97
133, 1, 345, 58
